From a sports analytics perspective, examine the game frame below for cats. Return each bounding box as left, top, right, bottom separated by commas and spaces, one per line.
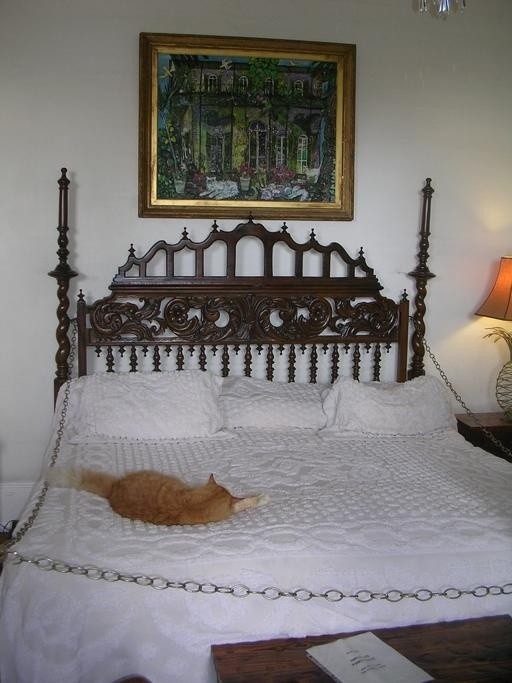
41, 462, 273, 528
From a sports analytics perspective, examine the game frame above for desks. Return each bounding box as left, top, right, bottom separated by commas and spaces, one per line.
211, 614, 512, 683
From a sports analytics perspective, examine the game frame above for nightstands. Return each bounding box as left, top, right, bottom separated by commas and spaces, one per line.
455, 411, 512, 463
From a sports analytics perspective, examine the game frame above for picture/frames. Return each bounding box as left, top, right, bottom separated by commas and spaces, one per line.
136, 30, 358, 222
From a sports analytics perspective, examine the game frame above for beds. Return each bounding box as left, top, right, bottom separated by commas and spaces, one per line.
0, 165, 512, 683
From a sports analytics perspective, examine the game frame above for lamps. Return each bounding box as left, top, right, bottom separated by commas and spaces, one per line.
411, 0, 470, 20
473, 254, 512, 418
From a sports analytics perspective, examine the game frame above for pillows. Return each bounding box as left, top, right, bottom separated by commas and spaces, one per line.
320, 376, 460, 437
73, 375, 225, 440
219, 374, 326, 434
